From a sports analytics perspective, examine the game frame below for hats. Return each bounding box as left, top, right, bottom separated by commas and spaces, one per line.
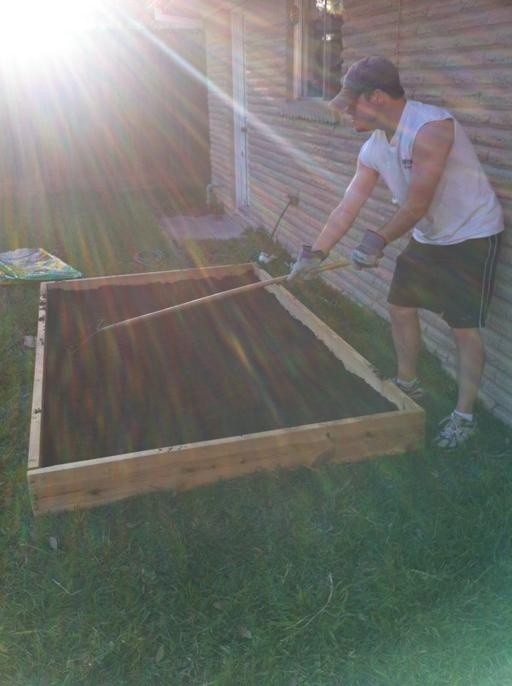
329, 54, 401, 110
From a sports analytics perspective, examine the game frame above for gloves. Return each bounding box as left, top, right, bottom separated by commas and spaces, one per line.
287, 246, 327, 284
352, 229, 386, 272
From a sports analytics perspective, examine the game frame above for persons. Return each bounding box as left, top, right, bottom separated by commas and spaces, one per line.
284, 54, 507, 452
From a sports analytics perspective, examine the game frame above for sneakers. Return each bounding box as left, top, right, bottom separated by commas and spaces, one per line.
394, 377, 426, 402
431, 412, 480, 452
